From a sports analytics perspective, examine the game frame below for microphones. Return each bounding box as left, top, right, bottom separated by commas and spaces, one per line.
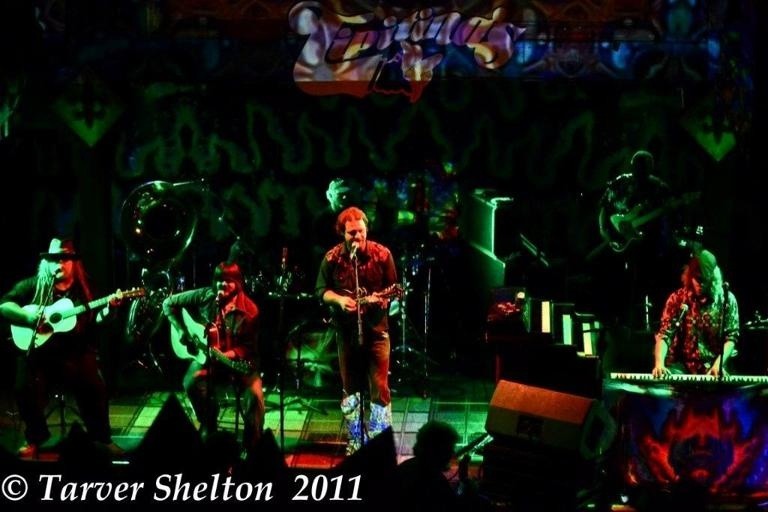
673, 301, 690, 329
348, 240, 361, 260
721, 277, 734, 303
216, 280, 226, 301
52, 266, 61, 279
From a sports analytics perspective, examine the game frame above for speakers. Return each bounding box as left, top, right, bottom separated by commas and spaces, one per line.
484, 379, 618, 459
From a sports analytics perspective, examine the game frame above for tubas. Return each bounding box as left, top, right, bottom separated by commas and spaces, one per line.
119, 181, 197, 342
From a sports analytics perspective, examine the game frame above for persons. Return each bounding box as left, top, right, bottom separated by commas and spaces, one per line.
597, 149, 684, 246
161, 260, 265, 455
0, 234, 126, 458
649, 247, 741, 381
0, 420, 481, 512
312, 205, 399, 457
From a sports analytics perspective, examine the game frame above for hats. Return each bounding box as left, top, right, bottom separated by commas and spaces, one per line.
689, 248, 718, 283
38, 236, 85, 261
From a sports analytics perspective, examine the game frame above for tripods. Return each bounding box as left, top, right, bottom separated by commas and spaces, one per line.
264, 326, 328, 415
391, 256, 460, 391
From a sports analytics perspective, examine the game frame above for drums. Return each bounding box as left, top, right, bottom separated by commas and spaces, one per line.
280, 315, 343, 395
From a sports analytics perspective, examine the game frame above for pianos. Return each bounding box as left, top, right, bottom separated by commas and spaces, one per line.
604, 371, 768, 394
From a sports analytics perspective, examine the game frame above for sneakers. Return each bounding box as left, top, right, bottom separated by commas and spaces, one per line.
103, 442, 127, 458
17, 431, 52, 459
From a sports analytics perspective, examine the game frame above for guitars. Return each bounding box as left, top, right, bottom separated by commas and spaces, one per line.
606, 190, 699, 253
10, 287, 145, 351
170, 305, 257, 377
340, 282, 405, 316
